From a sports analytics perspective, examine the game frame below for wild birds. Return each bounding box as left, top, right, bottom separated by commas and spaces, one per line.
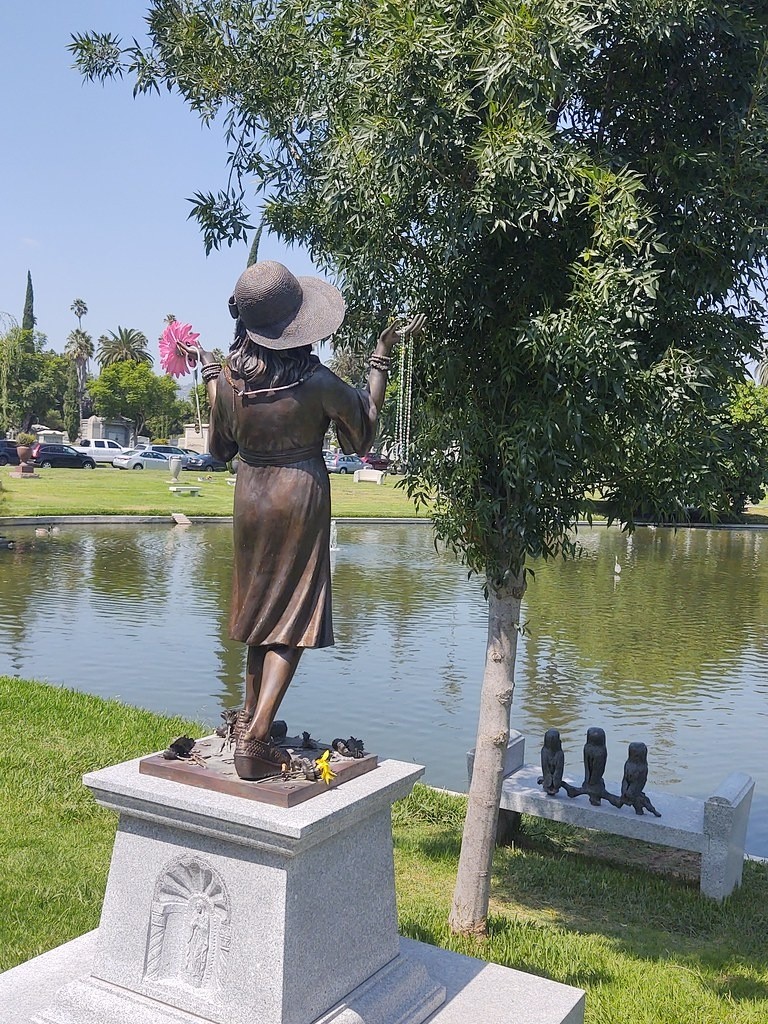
614, 556, 622, 574
35, 524, 61, 534
540, 727, 648, 815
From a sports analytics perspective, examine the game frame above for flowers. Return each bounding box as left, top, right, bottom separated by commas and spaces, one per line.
159, 319, 201, 379
258, 758, 319, 783
315, 749, 337, 786
332, 736, 364, 758
163, 736, 207, 769
215, 709, 238, 752
280, 731, 317, 749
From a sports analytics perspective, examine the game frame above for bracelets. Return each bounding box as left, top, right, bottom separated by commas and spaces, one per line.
201, 363, 222, 381
368, 354, 391, 371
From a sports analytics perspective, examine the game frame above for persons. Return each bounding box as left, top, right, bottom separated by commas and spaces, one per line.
177, 261, 428, 779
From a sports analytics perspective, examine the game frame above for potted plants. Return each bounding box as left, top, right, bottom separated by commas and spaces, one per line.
16, 432, 36, 466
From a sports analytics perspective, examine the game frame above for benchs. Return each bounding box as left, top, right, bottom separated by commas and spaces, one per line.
169, 487, 202, 497
467, 730, 755, 901
354, 469, 384, 485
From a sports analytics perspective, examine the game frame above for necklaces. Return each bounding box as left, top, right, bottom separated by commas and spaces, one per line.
393, 326, 414, 466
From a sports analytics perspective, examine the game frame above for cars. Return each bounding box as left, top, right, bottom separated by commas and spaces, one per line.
355, 452, 395, 470
28, 443, 96, 470
133, 443, 194, 469
112, 450, 170, 470
325, 454, 369, 474
186, 454, 227, 472
388, 462, 408, 475
0, 440, 20, 465
181, 448, 199, 456
321, 448, 333, 460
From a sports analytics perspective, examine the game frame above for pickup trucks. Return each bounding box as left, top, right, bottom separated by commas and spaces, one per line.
63, 438, 134, 468
